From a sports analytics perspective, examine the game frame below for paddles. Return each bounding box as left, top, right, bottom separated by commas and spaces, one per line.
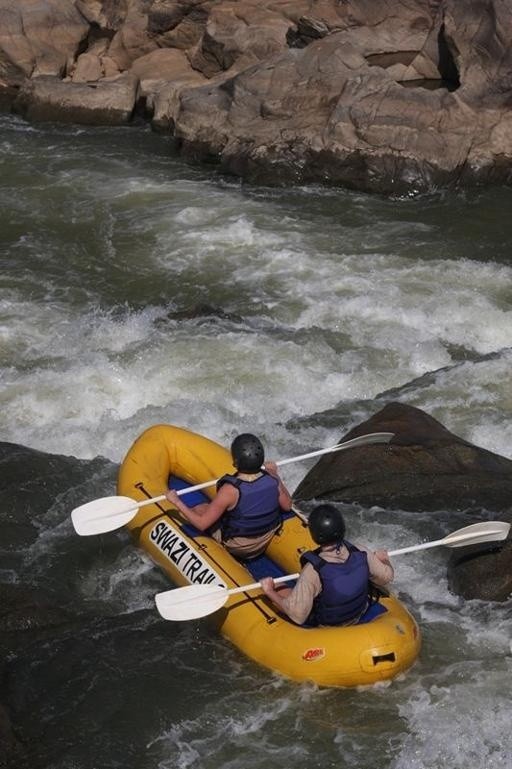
154, 522, 512, 621
70, 432, 396, 537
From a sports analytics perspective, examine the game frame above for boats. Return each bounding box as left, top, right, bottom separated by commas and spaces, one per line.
117, 423, 422, 689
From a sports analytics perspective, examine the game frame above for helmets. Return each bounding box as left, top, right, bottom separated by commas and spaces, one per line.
308, 504, 345, 544
231, 433, 264, 471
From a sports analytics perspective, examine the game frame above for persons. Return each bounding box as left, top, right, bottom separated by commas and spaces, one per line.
165, 431, 293, 560
258, 503, 395, 629
